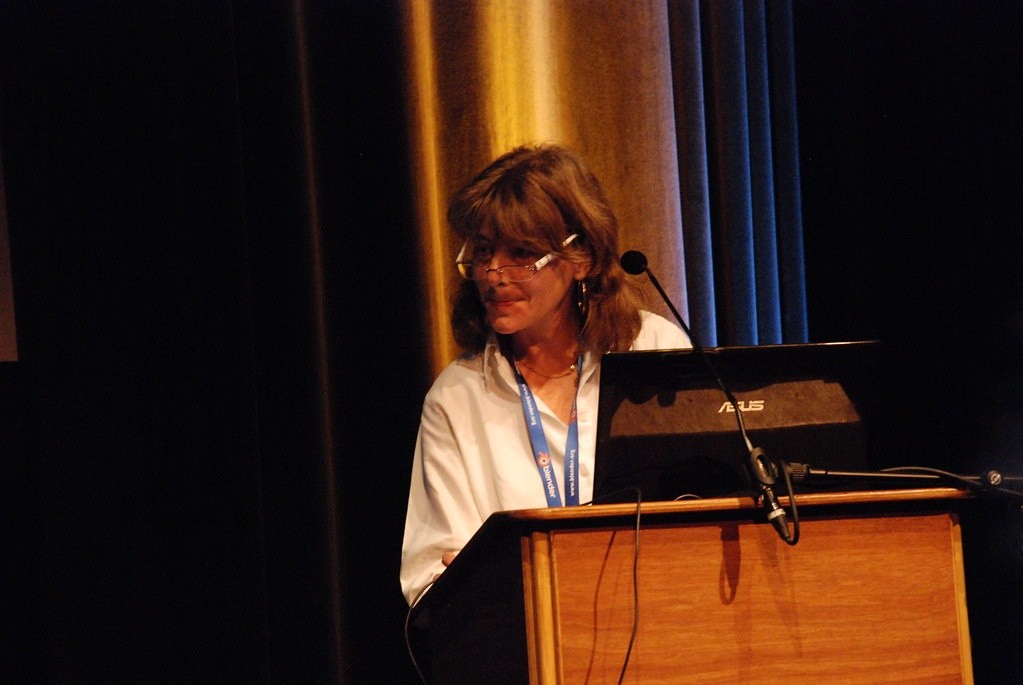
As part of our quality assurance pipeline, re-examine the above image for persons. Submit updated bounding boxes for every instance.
[399,147,693,608]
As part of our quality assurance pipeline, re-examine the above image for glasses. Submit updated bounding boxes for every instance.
[455,233,582,282]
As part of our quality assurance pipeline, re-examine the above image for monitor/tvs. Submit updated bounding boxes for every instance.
[589,339,884,507]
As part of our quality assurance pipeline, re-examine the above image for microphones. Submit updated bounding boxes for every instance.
[618,250,793,544]
